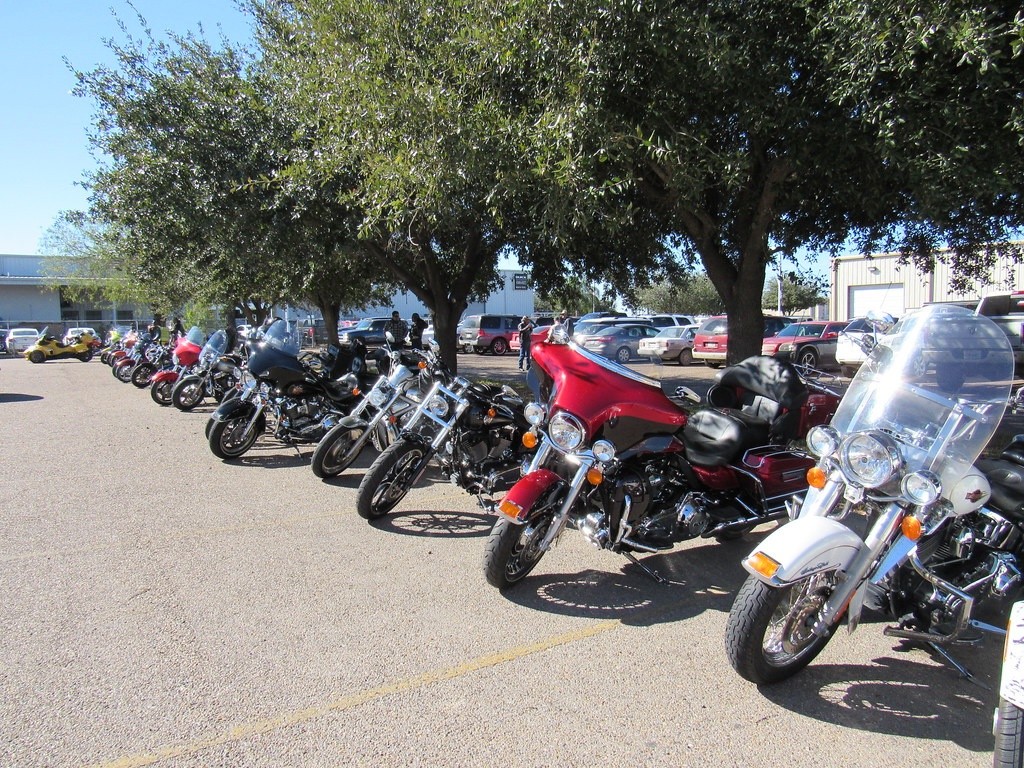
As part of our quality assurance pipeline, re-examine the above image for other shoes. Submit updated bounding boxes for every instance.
[519,368,524,372]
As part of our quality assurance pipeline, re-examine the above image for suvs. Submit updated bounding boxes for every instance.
[338,317,411,344]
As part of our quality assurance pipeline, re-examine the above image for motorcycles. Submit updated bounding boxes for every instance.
[22,326,102,363]
[95,304,1024,767]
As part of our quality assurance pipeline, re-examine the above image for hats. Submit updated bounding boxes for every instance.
[521,316,531,321]
[560,310,568,315]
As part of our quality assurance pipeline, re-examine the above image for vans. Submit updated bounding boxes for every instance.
[690,313,796,370]
[458,313,538,357]
[293,318,328,343]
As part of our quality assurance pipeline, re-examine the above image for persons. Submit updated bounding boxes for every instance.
[409,313,428,350]
[544,309,574,344]
[383,311,409,351]
[518,315,533,371]
[147,316,186,346]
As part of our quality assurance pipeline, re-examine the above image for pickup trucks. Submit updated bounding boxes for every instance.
[920,290,1024,391]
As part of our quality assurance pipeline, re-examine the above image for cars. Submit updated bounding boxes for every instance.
[834,314,929,382]
[636,324,705,366]
[1,328,41,355]
[762,321,849,376]
[583,323,673,364]
[509,325,552,350]
[60,327,103,350]
[237,323,253,339]
